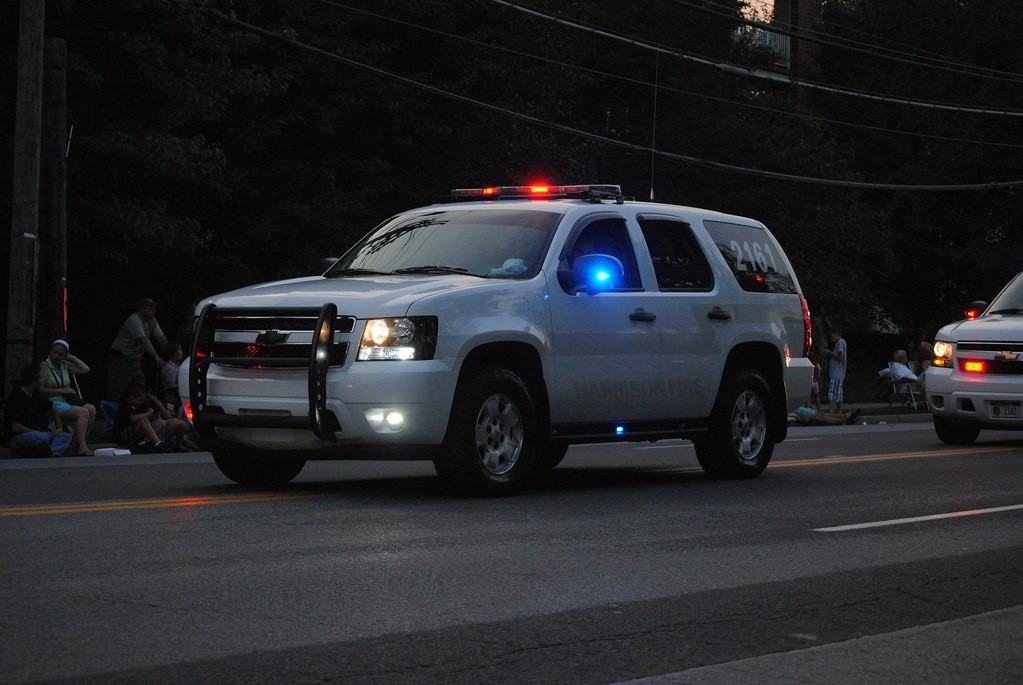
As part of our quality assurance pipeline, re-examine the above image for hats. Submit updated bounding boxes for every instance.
[139,298,159,308]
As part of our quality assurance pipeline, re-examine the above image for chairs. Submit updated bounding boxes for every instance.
[100,399,122,432]
[888,361,930,413]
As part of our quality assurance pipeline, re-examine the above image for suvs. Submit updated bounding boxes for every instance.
[177,185,816,501]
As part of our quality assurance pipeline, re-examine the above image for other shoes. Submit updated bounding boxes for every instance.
[174,446,193,453]
[846,408,861,424]
[154,439,172,453]
[136,443,154,454]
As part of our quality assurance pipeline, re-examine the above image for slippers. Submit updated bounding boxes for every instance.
[77,450,94,456]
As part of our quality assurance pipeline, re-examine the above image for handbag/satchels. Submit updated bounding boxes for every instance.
[64,393,85,406]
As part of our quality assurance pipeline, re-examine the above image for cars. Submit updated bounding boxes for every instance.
[925,271,1023,446]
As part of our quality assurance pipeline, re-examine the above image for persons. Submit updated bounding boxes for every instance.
[3,298,209,458]
[784,304,933,423]
[492,237,609,294]
